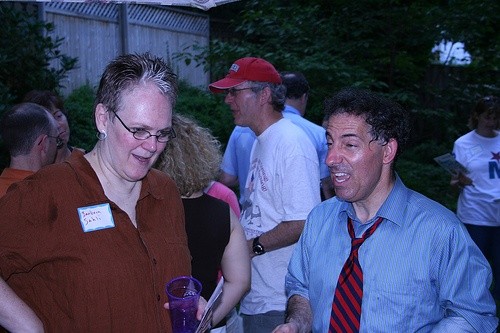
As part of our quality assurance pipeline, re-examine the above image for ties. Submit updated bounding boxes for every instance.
[328,216,383,333]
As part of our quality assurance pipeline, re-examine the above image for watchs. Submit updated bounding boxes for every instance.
[252,237,265,255]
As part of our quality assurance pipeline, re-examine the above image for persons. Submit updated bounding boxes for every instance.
[1,54,335,333]
[272,88,500,333]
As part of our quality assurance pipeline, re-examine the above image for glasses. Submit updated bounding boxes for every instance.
[37,135,63,146]
[109,106,177,143]
[478,96,500,110]
[226,86,253,95]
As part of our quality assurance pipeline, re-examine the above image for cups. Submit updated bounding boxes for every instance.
[166,276,201,333]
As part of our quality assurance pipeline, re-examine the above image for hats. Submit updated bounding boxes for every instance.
[209,57,283,94]
